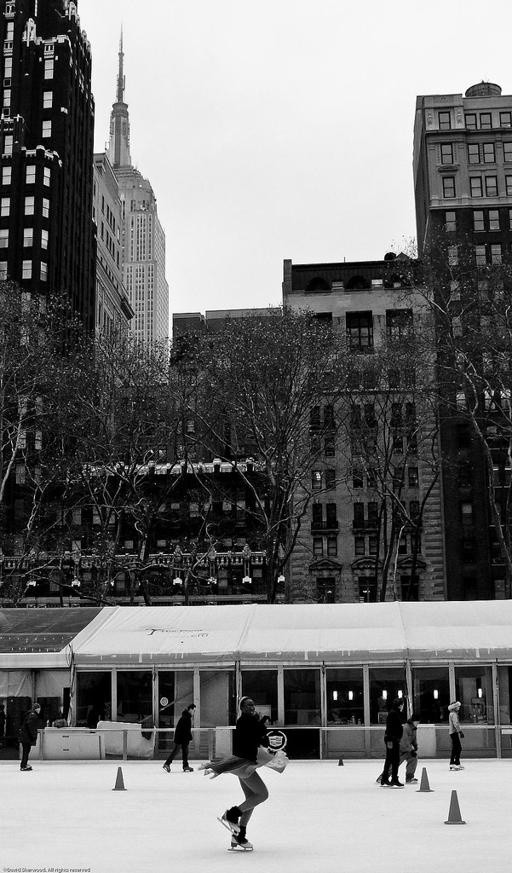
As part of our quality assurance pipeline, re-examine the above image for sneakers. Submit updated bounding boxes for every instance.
[163,763,171,771]
[449,763,455,768]
[183,765,193,770]
[20,763,32,770]
[406,777,418,783]
[454,763,464,769]
[376,776,383,783]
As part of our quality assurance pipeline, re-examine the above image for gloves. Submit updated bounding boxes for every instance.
[459,731,464,739]
[412,746,417,752]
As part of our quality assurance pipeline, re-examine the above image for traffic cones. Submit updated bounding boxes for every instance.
[443,788,466,824]
[109,764,128,790]
[414,766,436,792]
[336,753,347,766]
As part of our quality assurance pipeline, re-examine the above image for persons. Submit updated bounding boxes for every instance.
[378,697,405,787]
[369,695,379,723]
[164,702,197,771]
[310,707,335,725]
[84,695,109,729]
[376,710,420,783]
[16,702,43,769]
[327,707,343,721]
[222,695,270,849]
[446,700,465,769]
[0,703,8,739]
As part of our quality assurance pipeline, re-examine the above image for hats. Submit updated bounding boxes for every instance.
[32,703,40,710]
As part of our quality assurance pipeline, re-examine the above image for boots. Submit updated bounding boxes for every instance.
[223,806,243,833]
[390,775,405,786]
[380,773,392,785]
[230,826,254,848]
